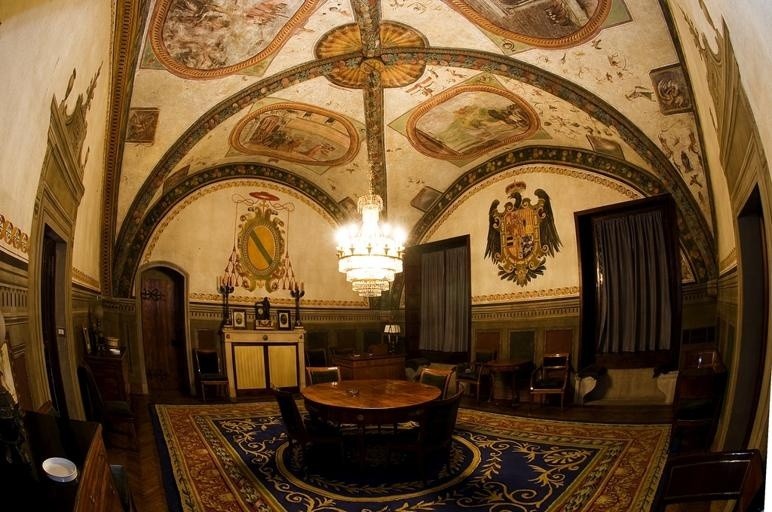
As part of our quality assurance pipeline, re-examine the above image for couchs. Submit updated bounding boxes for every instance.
[572,351,680,407]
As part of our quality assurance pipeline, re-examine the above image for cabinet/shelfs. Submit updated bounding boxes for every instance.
[660,450,766,511]
[83,346,131,414]
[0,410,127,512]
[672,351,727,453]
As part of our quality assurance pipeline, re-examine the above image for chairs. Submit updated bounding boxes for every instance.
[305,366,341,390]
[394,367,459,439]
[458,350,497,401]
[384,386,465,480]
[192,350,229,401]
[77,367,138,452]
[529,353,570,409]
[276,391,341,479]
[305,348,341,387]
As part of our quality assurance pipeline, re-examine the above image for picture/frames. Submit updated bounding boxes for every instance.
[82,328,91,353]
[233,309,246,329]
[277,310,291,330]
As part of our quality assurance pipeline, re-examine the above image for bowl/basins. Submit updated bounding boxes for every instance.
[41,455,79,482]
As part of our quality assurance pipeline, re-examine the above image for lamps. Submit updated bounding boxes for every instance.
[384,324,400,353]
[95,305,104,351]
[334,70,407,298]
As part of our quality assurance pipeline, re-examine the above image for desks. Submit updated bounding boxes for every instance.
[300,379,442,448]
[327,353,400,380]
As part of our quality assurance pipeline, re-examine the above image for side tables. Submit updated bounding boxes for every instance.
[489,357,531,407]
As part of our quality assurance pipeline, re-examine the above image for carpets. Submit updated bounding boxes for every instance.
[147,398,672,511]
